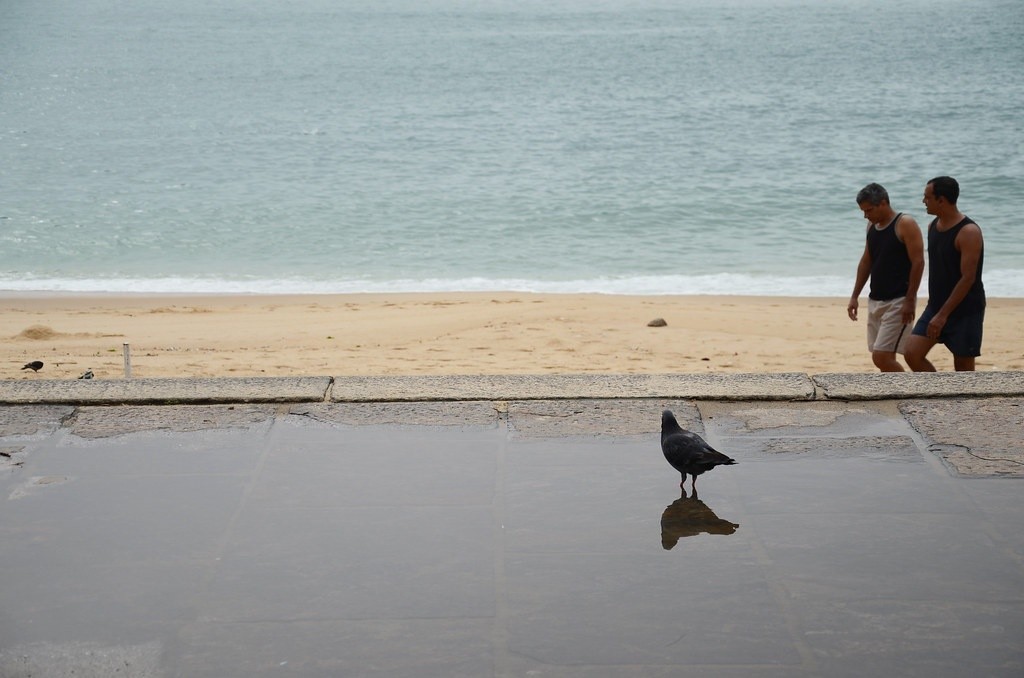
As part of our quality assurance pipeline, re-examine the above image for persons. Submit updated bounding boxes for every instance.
[846,182,925,373]
[908,175,988,371]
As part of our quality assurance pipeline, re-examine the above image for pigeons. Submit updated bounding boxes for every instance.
[21,361,44,374]
[660,409,742,492]
[77,367,95,380]
